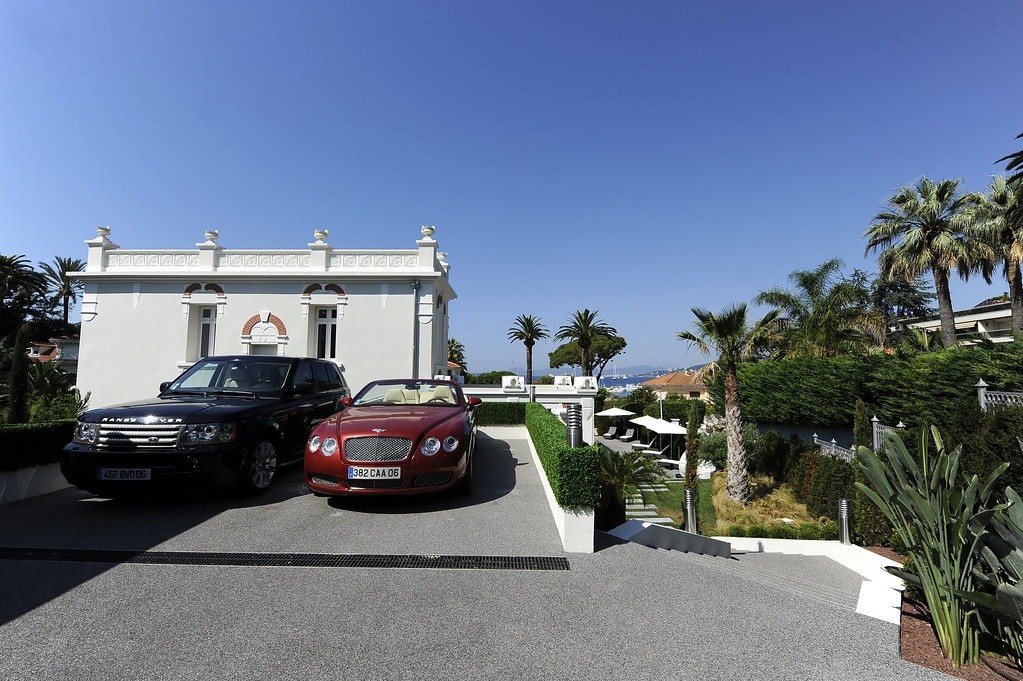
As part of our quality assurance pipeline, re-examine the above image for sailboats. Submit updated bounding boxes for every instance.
[598,365,643,392]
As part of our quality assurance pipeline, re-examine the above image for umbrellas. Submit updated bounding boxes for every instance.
[629,414,687,459]
[595,406,637,426]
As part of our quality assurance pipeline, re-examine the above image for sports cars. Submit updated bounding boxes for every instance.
[302,378,485,498]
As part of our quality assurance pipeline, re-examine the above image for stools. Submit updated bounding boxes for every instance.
[657,458,680,470]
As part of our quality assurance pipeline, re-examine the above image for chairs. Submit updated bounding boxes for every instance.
[632,437,657,450]
[602,426,617,439]
[382,388,407,405]
[619,428,635,442]
[642,445,671,459]
[430,385,457,405]
[222,369,250,389]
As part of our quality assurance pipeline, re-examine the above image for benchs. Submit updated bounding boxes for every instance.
[402,390,435,404]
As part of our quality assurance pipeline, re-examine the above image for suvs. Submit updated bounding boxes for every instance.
[59,354,354,506]
